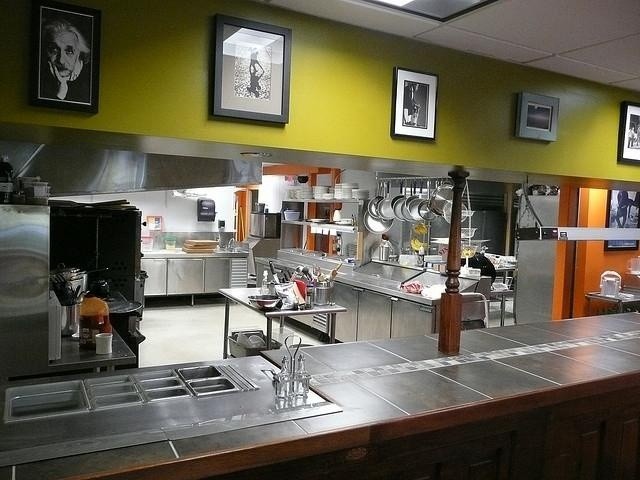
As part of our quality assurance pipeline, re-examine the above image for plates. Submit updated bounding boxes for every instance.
[346,243,357,258]
[307,218,330,223]
[286,181,369,200]
[430,238,449,245]
[423,256,446,263]
[335,217,353,226]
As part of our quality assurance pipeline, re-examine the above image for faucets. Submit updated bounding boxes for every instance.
[228,237,236,248]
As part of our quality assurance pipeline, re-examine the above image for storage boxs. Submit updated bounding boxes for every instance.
[228,333,281,358]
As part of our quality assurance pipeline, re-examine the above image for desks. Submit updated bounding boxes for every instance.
[0,319,137,383]
[494,266,516,309]
[489,289,514,327]
[0,312,640,480]
[218,286,347,359]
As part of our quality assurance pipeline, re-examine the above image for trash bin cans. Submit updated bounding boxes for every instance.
[140,271,149,309]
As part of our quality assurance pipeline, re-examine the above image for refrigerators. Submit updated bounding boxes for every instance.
[512,193,561,325]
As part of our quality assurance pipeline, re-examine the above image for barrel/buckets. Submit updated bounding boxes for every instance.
[599,269,622,296]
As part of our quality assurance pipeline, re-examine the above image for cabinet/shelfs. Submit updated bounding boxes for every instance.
[584,289,640,317]
[142,257,229,295]
[280,198,370,233]
[257,263,436,344]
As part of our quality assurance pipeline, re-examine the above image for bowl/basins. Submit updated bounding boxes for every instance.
[460,227,478,239]
[460,246,479,258]
[248,335,265,348]
[461,210,476,216]
[284,211,301,221]
[236,332,253,348]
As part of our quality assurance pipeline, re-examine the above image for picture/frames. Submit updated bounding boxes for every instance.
[604,190,639,251]
[211,13,292,124]
[515,90,560,142]
[617,100,640,166]
[390,66,439,141]
[28,0,102,113]
[146,216,162,231]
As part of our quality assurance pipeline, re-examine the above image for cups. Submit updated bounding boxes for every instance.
[626,257,640,274]
[59,301,80,335]
[314,277,335,306]
[95,333,114,356]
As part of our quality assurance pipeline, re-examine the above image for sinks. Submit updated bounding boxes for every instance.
[228,249,240,253]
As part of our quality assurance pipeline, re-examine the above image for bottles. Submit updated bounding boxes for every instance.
[304,284,316,310]
[295,354,313,396]
[260,270,271,293]
[1,155,15,206]
[273,356,293,402]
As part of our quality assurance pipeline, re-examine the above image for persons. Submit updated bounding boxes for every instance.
[628,126,640,148]
[250,51,266,72]
[403,82,422,126]
[40,18,92,103]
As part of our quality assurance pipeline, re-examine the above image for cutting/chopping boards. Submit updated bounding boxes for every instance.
[182,239,219,254]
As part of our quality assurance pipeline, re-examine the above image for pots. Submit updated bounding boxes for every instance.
[360,173,469,235]
[246,293,288,311]
[49,260,110,295]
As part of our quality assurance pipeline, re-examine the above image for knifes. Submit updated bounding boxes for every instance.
[280,269,295,285]
[268,260,281,285]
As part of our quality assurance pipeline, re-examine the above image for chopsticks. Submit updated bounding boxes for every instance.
[48,273,83,305]
